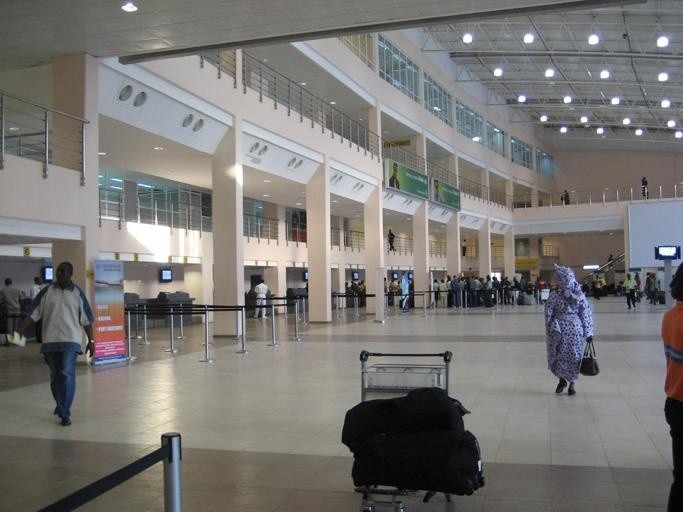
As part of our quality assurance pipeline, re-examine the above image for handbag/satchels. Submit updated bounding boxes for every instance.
[341,388,484,495]
[580,357,599,375]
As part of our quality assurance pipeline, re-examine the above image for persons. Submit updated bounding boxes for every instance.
[384,276,414,308]
[433,179,443,203]
[387,228,396,252]
[640,176,649,199]
[388,162,399,189]
[346,281,366,306]
[29,276,49,343]
[582,255,613,300]
[433,274,553,307]
[542,264,599,397]
[462,238,466,256]
[254,279,268,319]
[14,262,95,427]
[0,278,25,347]
[560,189,570,205]
[660,260,683,512]
[623,272,657,309]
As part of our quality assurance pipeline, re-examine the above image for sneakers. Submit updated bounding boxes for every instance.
[556,378,567,392]
[569,383,576,394]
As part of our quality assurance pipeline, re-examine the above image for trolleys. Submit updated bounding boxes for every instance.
[352,350,454,511]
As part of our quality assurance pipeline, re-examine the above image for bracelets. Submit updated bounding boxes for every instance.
[89,339,94,343]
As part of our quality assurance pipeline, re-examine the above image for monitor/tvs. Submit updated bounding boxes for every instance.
[392,272,398,280]
[41,265,53,284]
[159,268,172,282]
[303,272,308,281]
[353,272,359,280]
[408,272,412,280]
[655,245,681,260]
